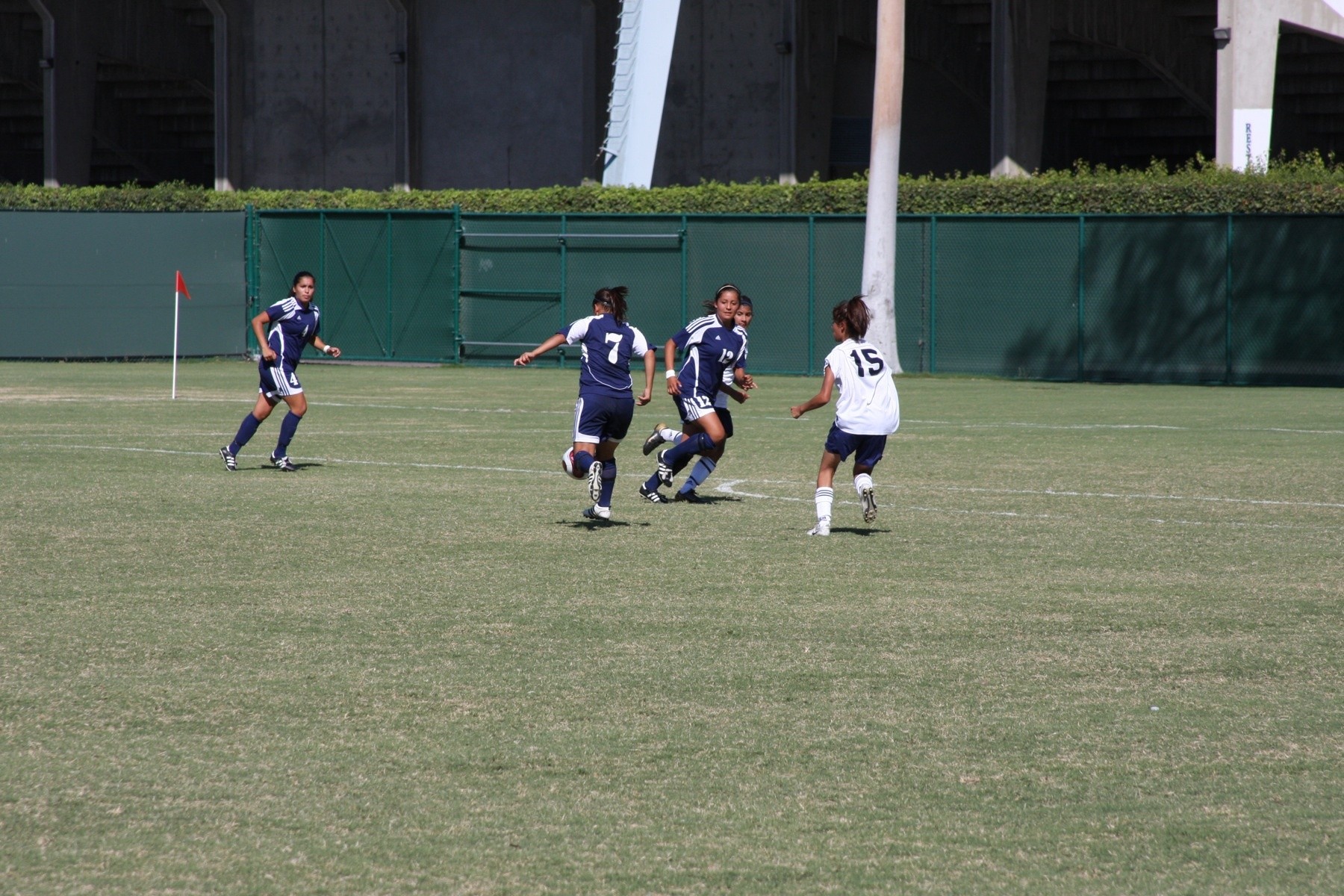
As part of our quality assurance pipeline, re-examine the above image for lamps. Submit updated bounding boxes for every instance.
[1213,27,1231,39]
[389,51,405,63]
[775,40,793,55]
[39,56,54,68]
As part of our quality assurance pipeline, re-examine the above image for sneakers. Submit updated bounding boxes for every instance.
[582,502,611,521]
[655,448,674,488]
[221,445,238,473]
[675,488,712,504]
[644,423,669,456]
[808,522,831,537]
[588,461,603,501]
[639,481,668,505]
[271,450,296,471]
[860,484,878,523]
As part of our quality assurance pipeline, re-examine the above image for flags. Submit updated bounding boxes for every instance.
[175,270,191,299]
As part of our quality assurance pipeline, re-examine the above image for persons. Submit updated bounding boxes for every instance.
[219,271,341,473]
[514,286,657,520]
[790,295,900,537]
[514,283,758,522]
